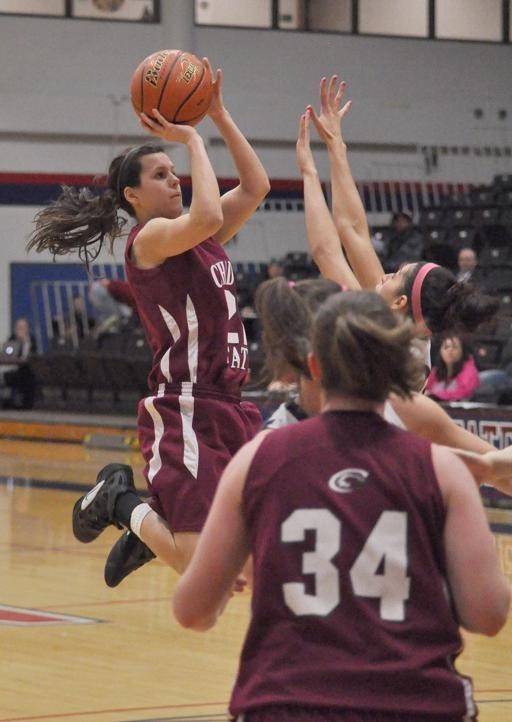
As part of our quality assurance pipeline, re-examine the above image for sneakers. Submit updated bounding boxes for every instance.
[72,463,137,543]
[104,529,158,588]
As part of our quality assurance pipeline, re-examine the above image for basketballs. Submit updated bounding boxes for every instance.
[130,48,212,125]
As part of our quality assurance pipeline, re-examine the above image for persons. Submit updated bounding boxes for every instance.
[425,329,472,397]
[254,269,510,488]
[22,54,269,586]
[168,299,509,721]
[383,205,426,272]
[52,293,93,337]
[446,238,487,286]
[234,286,259,346]
[5,318,36,362]
[294,58,493,382]
